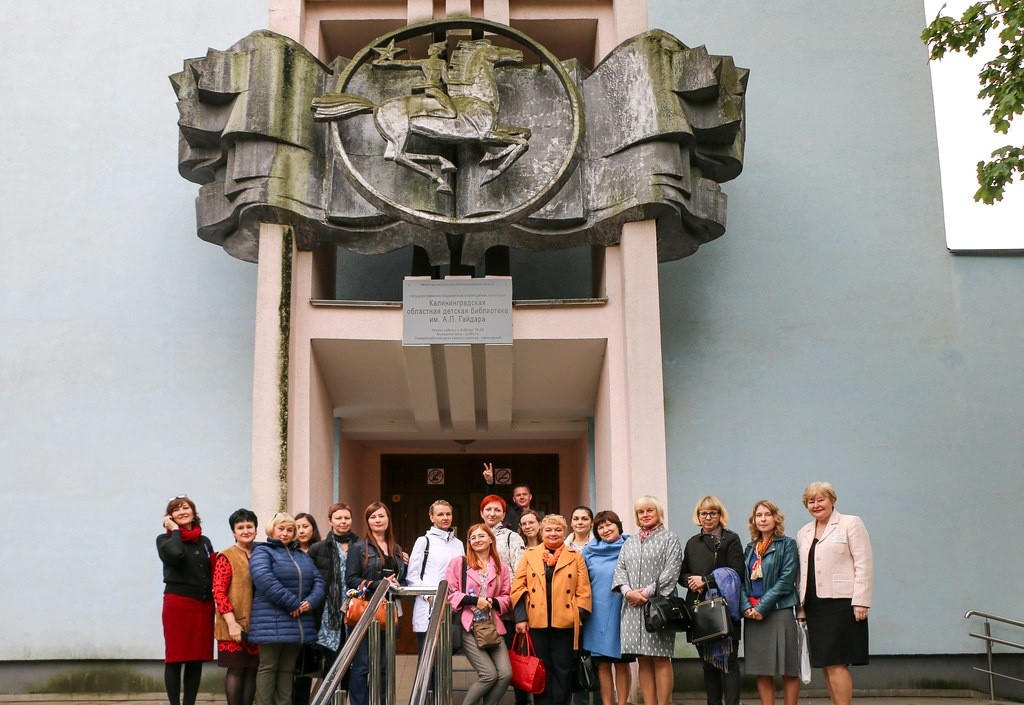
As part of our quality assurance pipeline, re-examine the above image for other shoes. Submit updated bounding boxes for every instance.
[451,611,463,656]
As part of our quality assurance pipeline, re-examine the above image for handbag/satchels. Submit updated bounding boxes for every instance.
[644,579,694,633]
[399,536,430,608]
[507,630,547,695]
[472,619,501,650]
[692,576,734,645]
[571,655,598,693]
[345,581,399,630]
[795,620,812,686]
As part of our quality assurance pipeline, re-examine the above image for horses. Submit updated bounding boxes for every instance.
[310,39,531,193]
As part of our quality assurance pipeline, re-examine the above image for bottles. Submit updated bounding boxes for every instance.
[468,589,487,619]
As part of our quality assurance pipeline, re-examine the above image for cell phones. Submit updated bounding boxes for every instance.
[381,569,393,578]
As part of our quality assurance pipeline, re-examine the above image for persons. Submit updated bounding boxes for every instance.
[309,503,409,699]
[345,502,405,705]
[741,499,800,705]
[445,524,512,705]
[518,510,543,550]
[678,495,744,705]
[249,514,324,705]
[406,501,464,705]
[611,496,682,705]
[156,497,214,705]
[582,511,630,705]
[480,495,531,705]
[292,513,321,705]
[563,506,603,705]
[372,41,475,118]
[213,509,259,705]
[511,514,592,705]
[797,482,873,705]
[483,462,545,533]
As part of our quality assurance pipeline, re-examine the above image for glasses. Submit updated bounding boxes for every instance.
[168,494,187,503]
[468,534,486,542]
[697,512,720,518]
